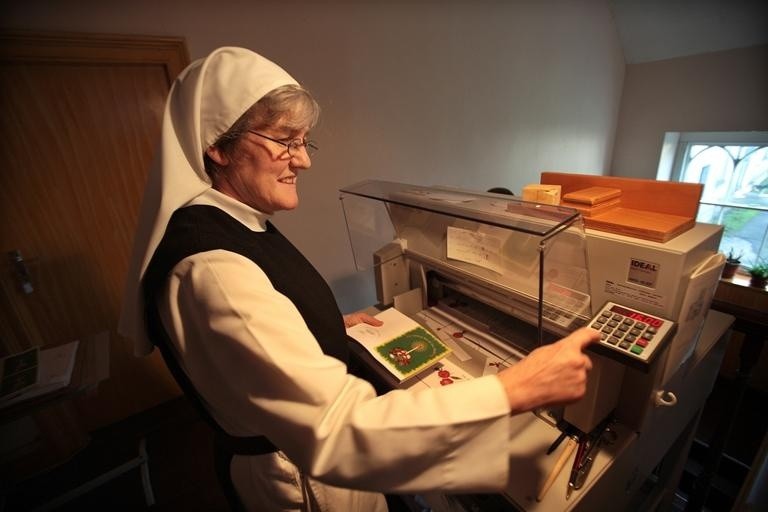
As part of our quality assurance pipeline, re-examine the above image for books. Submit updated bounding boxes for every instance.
[1,344,44,401]
[1,339,82,408]
[344,306,453,384]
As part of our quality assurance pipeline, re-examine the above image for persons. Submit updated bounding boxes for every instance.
[116,46,607,511]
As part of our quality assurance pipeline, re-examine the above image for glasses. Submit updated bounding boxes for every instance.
[248,131,320,161]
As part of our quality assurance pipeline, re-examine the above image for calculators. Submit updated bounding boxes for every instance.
[586,301,677,363]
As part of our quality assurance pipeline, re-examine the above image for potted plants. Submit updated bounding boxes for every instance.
[724,248,768,291]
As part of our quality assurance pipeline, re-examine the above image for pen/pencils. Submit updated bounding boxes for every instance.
[536,424,619,502]
